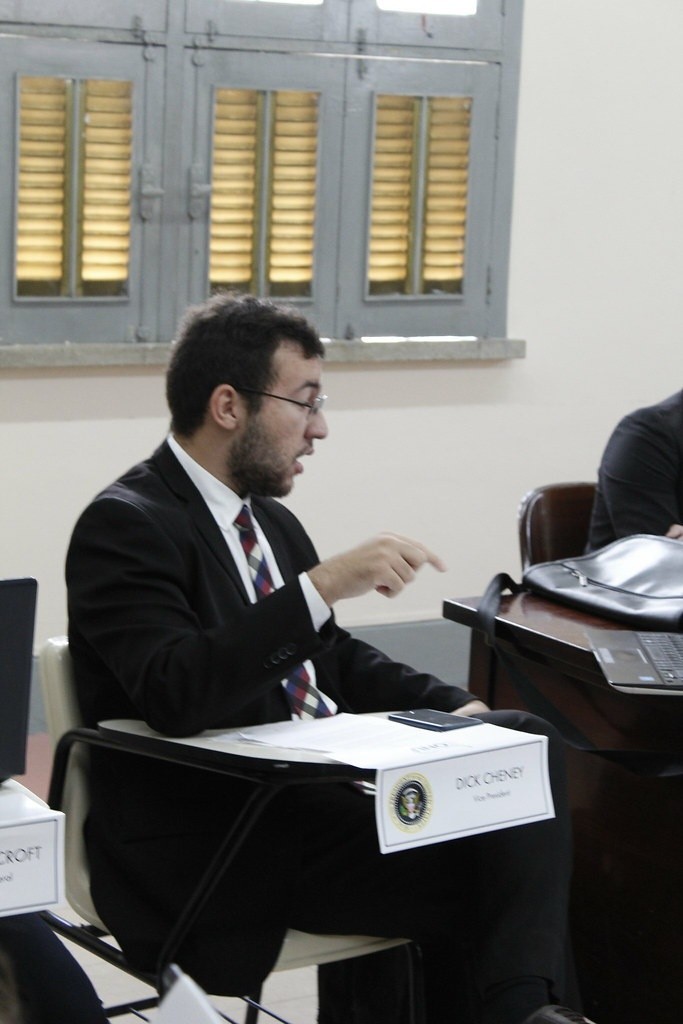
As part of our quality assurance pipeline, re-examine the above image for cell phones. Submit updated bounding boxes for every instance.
[388,707,483,733]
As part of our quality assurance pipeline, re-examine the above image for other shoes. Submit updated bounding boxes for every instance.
[522,1005,598,1024]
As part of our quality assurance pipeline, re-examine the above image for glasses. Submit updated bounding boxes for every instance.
[233,384,328,415]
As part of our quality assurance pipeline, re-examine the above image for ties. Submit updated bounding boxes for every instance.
[232,504,334,726]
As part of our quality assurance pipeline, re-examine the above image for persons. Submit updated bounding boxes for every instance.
[62,293,573,1024]
[581,388,683,554]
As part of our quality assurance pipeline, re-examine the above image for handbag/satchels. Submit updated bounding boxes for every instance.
[523,534,683,633]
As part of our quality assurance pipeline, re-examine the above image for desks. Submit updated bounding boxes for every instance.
[442,591,682,1022]
[45,704,409,972]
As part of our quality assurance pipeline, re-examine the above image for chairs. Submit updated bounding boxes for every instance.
[0,579,161,1024]
[520,483,598,576]
[37,636,436,1024]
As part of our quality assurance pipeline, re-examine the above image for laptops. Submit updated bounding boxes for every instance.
[583,625,683,696]
